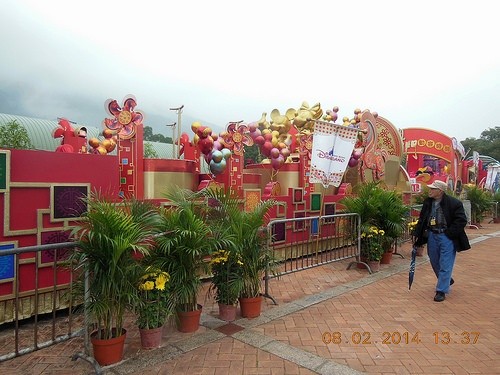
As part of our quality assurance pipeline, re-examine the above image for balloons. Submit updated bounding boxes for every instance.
[363,109,378,118]
[260,141,272,157]
[192,122,212,138]
[342,118,356,129]
[55,145,73,153]
[353,150,362,159]
[353,109,362,123]
[204,148,232,174]
[323,106,339,122]
[269,148,290,169]
[190,134,199,146]
[348,157,358,167]
[89,138,99,147]
[249,123,271,143]
[96,128,117,154]
[200,134,225,153]
[219,131,228,137]
[271,132,287,150]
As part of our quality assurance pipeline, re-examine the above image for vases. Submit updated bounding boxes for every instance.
[416,245,424,257]
[137,323,163,350]
[368,262,382,272]
[217,301,237,323]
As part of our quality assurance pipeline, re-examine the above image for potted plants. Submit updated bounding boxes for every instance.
[327,177,412,269]
[463,182,500,229]
[56,184,287,367]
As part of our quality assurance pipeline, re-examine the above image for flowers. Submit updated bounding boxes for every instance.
[135,265,174,330]
[359,224,386,262]
[407,218,427,248]
[209,248,243,304]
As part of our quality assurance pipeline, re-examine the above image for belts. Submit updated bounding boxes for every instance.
[427,226,447,234]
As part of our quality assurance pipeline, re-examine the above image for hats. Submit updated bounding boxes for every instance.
[427,179,447,191]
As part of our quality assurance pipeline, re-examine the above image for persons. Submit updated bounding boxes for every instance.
[414,180,471,301]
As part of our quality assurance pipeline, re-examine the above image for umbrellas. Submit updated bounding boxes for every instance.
[409,245,416,291]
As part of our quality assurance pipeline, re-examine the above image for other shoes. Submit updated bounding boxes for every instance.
[450,277,454,285]
[434,291,445,302]
